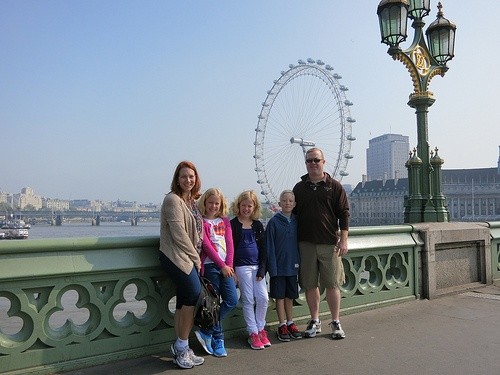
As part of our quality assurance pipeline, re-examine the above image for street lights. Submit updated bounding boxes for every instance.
[376,1,456,224]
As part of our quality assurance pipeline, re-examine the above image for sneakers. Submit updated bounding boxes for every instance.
[194,328,214,355]
[248,330,271,349]
[277,323,302,342]
[212,338,228,357]
[169,343,204,368]
[330,321,345,340]
[304,319,321,337]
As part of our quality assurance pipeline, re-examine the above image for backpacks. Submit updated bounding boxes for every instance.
[193,274,221,330]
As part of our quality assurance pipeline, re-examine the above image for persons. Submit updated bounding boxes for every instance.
[292,147,351,339]
[194,189,239,356]
[230,190,272,349]
[266,189,303,342]
[160,161,205,368]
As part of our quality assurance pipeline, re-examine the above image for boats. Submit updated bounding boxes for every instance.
[0,214,32,239]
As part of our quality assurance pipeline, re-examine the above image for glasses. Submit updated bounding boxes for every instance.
[306,158,323,163]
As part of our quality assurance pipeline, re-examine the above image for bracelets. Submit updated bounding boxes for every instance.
[195,261,201,267]
